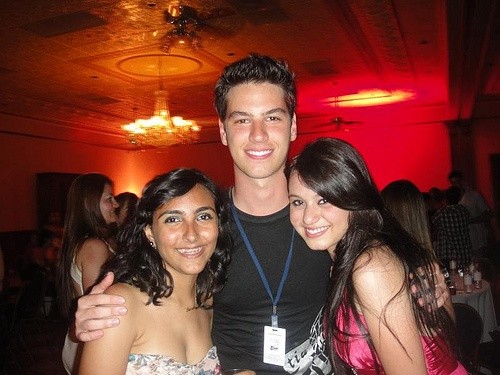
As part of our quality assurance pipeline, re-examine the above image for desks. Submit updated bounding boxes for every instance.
[451,279,497,343]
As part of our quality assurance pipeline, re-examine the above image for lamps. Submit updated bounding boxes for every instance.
[119,57,201,149]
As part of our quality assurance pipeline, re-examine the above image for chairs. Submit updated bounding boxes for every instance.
[452,303,483,356]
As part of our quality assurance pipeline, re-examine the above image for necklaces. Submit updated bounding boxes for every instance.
[172,281,196,312]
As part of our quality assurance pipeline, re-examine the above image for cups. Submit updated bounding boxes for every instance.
[444,258,483,295]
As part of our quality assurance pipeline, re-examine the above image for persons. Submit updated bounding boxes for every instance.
[61,173,140,375]
[378,170,500,260]
[284,136,470,375]
[77,168,256,375]
[63,52,455,375]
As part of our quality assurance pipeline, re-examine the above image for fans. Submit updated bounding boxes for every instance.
[312,96,364,127]
